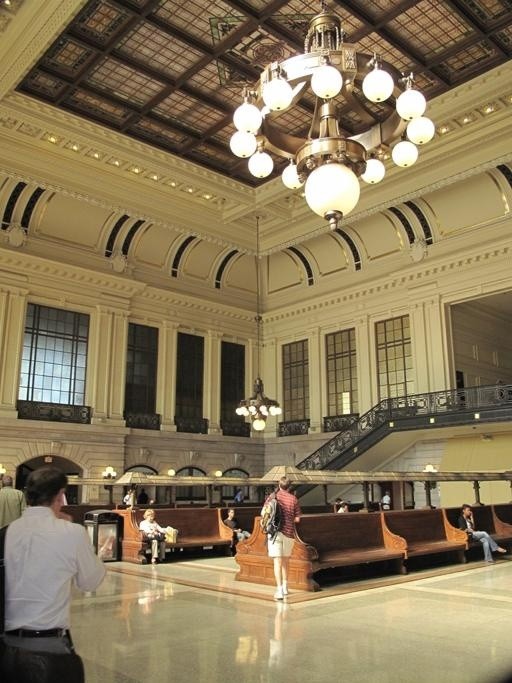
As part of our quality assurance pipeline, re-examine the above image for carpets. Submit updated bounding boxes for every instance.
[103,553,511,604]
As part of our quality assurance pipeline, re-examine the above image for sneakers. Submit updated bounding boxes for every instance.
[152,558,166,564]
[276,585,287,600]
[497,548,506,553]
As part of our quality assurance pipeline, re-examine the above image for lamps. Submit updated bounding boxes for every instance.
[103,465,116,503]
[235,213,283,431]
[423,464,436,504]
[215,470,223,477]
[168,469,175,477]
[228,0,436,231]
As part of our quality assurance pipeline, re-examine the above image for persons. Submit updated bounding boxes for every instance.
[139,507,168,564]
[123,488,132,505]
[334,497,342,512]
[458,503,508,562]
[259,474,302,600]
[1,473,29,529]
[380,490,391,510]
[138,487,149,503]
[234,486,246,502]
[225,507,252,542]
[1,465,108,681]
[129,489,137,508]
[337,501,349,513]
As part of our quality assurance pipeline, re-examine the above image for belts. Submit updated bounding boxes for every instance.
[7,629,59,638]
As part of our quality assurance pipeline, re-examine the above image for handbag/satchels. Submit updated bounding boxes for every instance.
[166,527,177,543]
[467,532,473,542]
[0,639,84,683]
[146,531,165,541]
[261,493,283,537]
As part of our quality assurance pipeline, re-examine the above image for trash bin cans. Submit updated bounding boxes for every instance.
[84,509,122,561]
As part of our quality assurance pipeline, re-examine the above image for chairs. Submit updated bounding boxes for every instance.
[230,504,510,590]
[116,504,407,565]
